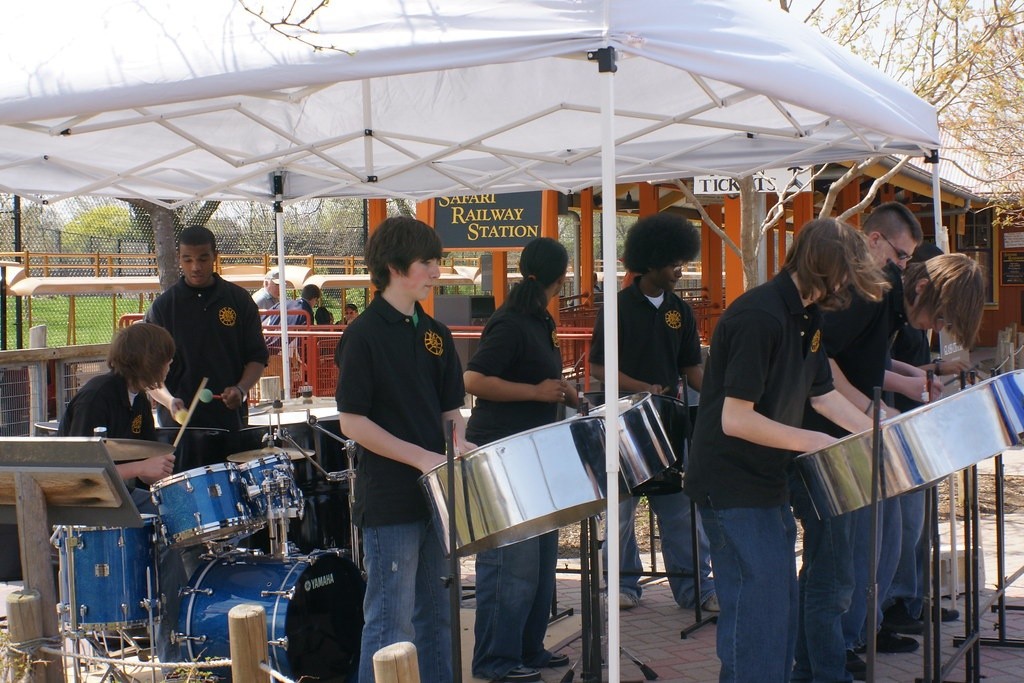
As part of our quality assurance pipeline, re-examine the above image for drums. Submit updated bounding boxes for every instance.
[791,367,1024,522]
[416,391,705,561]
[236,451,305,521]
[51,522,164,638]
[175,544,367,683]
[148,461,268,549]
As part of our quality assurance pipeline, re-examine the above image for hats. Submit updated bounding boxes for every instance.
[265,268,285,284]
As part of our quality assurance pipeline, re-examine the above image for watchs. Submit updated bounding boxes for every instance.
[234,385,248,404]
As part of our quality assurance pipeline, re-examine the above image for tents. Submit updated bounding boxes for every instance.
[0,0,940,683]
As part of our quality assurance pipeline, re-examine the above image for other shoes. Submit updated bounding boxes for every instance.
[704,595,719,610]
[884,605,923,634]
[844,649,867,679]
[604,594,637,607]
[919,601,958,620]
[875,632,918,653]
[506,666,540,681]
[546,653,569,666]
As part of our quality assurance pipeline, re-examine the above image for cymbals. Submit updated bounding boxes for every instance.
[93,436,177,461]
[244,395,340,427]
[226,446,316,463]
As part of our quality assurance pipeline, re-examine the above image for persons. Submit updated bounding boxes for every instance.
[251,266,358,362]
[591,214,721,615]
[684,206,987,683]
[335,217,480,683]
[144,225,270,474]
[56,323,175,486]
[463,242,579,683]
[592,272,602,292]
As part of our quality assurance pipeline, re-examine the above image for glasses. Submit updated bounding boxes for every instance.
[879,233,913,261]
[669,263,683,272]
[932,312,954,327]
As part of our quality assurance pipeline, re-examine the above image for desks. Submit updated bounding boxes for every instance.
[33,396,340,548]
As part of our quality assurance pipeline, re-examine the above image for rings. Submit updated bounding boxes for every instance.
[563,392,565,397]
